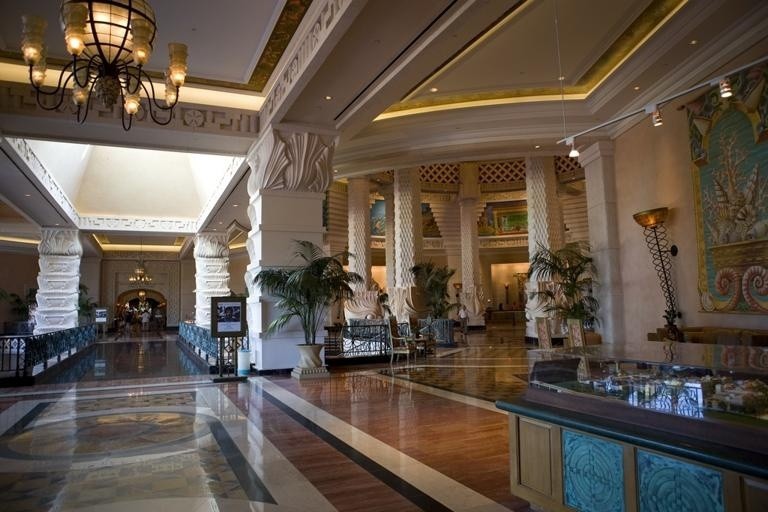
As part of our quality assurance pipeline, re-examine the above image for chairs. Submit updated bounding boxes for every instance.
[385,314,437,366]
[417,316,455,349]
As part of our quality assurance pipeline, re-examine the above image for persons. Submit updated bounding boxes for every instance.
[139,310,151,331]
[458,304,470,335]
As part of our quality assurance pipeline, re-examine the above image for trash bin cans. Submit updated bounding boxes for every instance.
[237,350,250,374]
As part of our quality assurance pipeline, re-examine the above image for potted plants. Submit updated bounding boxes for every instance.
[251,236,366,368]
[1,287,39,335]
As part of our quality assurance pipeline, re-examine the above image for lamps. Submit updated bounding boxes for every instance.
[18,0,191,131]
[718,78,732,98]
[651,108,663,126]
[128,238,154,286]
[632,205,681,338]
[568,142,579,158]
